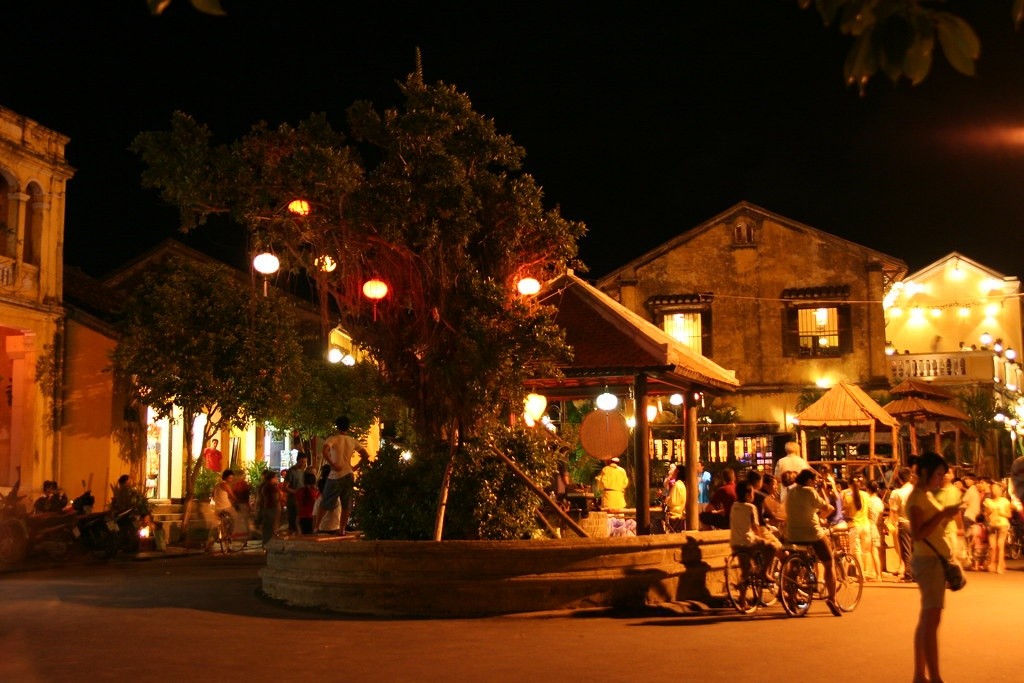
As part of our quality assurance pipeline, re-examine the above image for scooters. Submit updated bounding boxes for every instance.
[1,464,150,567]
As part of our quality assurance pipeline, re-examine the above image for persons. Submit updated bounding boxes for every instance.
[1012,435,1024,506]
[313,416,370,535]
[705,468,736,518]
[933,335,943,352]
[551,441,570,495]
[730,469,783,611]
[30,480,58,515]
[232,469,252,548]
[203,438,222,472]
[596,457,629,522]
[893,350,909,354]
[958,341,977,352]
[283,452,331,534]
[255,469,282,548]
[774,442,823,481]
[904,451,960,683]
[781,469,842,616]
[280,469,288,478]
[660,458,711,533]
[818,454,917,583]
[941,469,1011,575]
[205,468,239,552]
[111,474,134,530]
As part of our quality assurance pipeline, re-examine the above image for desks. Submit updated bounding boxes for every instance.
[601,506,663,519]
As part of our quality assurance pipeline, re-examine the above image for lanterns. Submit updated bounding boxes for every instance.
[328,345,355,366]
[597,382,684,427]
[518,271,541,306]
[253,247,279,298]
[363,277,388,322]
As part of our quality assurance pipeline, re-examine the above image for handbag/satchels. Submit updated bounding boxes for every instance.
[946,561,966,592]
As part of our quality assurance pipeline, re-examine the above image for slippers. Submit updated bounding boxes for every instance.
[825,599,841,616]
[788,599,801,614]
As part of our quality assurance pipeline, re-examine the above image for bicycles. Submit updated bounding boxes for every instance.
[217,500,249,555]
[778,517,865,618]
[724,532,792,615]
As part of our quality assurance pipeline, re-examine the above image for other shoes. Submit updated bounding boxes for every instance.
[737,600,752,609]
[756,573,776,583]
